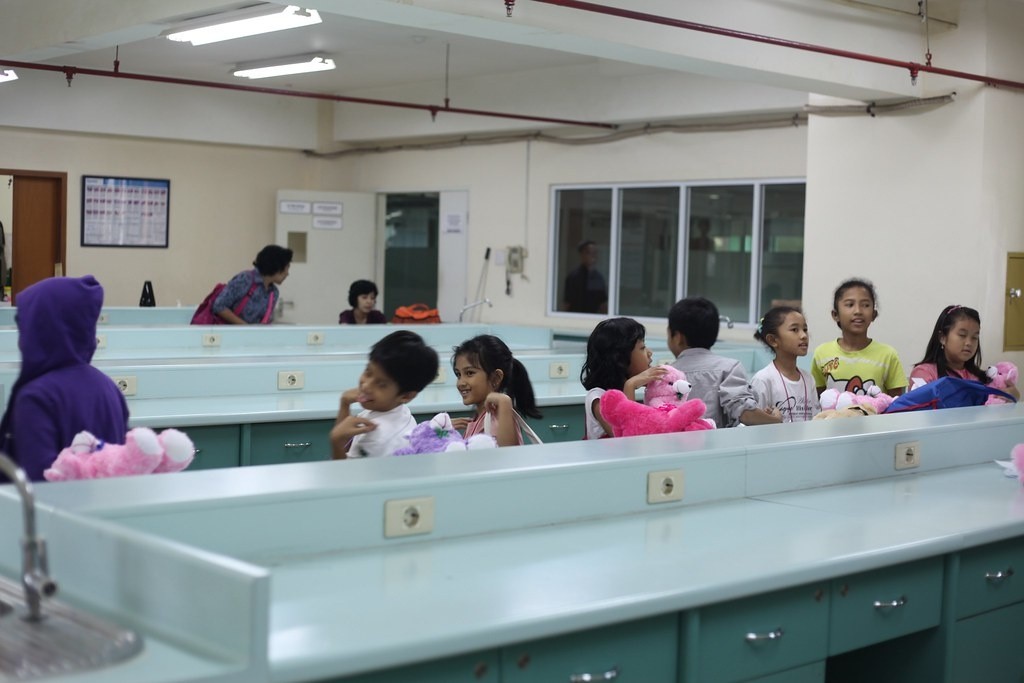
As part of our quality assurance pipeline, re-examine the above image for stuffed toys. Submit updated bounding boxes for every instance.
[1014,443,1024,487]
[600,389,716,437]
[812,403,878,419]
[819,385,898,414]
[393,412,497,457]
[984,362,1017,406]
[644,364,692,410]
[42,426,194,482]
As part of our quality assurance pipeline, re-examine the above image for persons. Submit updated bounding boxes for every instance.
[213,244,293,326]
[448,334,543,447]
[907,305,1020,404]
[580,317,669,438]
[329,329,440,459]
[667,297,782,428]
[339,280,387,324]
[749,305,822,422]
[0,274,129,485]
[811,280,910,398]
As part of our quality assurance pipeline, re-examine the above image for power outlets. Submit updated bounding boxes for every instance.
[382,498,434,537]
[96,334,107,348]
[894,441,921,469]
[201,334,221,347]
[549,363,570,379]
[647,469,683,504]
[307,332,325,345]
[658,358,673,368]
[108,375,137,396]
[432,365,448,383]
[97,315,107,324]
[278,371,303,389]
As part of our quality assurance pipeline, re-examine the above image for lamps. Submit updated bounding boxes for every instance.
[0,66,18,83]
[158,5,323,46]
[231,52,336,80]
[460,299,492,323]
[718,314,733,329]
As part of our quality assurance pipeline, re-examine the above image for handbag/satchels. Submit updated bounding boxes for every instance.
[190,270,255,325]
[880,376,1017,414]
[392,303,441,323]
[139,281,155,307]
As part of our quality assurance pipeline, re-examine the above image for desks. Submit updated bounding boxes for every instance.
[0,302,1024,683]
